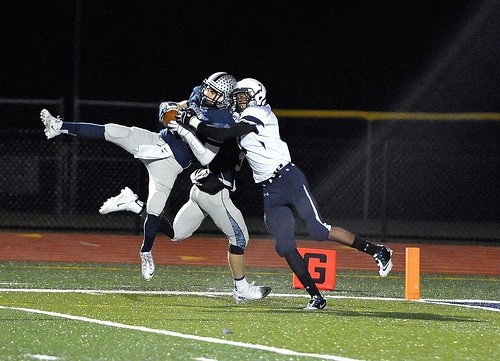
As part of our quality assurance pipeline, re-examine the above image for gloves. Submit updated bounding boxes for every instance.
[175,107,199,127]
[159,101,177,121]
[167,120,183,136]
[190,164,212,186]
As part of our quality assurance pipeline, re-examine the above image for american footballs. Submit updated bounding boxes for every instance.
[160,108,179,128]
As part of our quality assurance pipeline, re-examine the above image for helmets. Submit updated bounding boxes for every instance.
[203,72,237,101]
[231,78,266,106]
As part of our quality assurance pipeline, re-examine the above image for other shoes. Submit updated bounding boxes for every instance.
[99,187,138,214]
[233,280,272,305]
[303,294,327,310]
[373,243,394,277]
[40,109,62,139]
[139,240,155,282]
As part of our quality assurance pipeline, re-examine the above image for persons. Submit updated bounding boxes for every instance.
[39,71,393,310]
